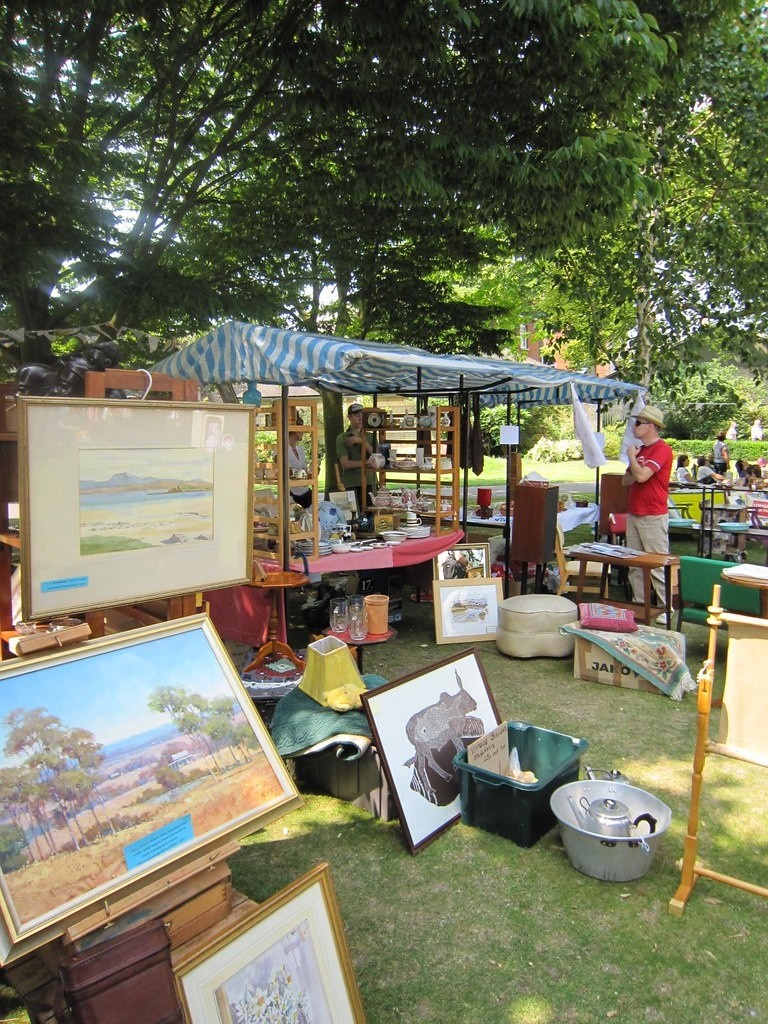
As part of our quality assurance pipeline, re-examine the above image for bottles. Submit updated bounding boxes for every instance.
[389,410,394,423]
[401,408,414,429]
[417,416,432,428]
[494,516,514,523]
[369,452,385,469]
[442,411,450,427]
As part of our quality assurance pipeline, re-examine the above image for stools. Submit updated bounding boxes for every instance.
[497,594,578,659]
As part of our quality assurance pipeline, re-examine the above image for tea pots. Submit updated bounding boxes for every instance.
[584,765,631,785]
[566,793,658,838]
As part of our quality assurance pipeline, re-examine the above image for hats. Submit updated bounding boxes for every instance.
[348,403,363,414]
[631,405,666,429]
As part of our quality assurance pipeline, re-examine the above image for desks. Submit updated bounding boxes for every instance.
[571,548,679,630]
[201,524,465,647]
[445,501,602,558]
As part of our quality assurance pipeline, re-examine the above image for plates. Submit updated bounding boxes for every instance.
[297,539,332,556]
[349,539,400,551]
[398,526,431,538]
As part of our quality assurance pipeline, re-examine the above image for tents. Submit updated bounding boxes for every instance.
[137,321,647,600]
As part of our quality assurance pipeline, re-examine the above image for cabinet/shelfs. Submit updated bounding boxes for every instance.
[252,400,319,564]
[668,482,768,566]
[360,406,460,536]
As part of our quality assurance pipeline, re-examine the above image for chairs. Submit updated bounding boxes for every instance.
[554,523,612,598]
[677,556,768,633]
[666,496,694,520]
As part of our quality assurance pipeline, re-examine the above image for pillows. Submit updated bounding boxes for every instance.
[577,601,639,632]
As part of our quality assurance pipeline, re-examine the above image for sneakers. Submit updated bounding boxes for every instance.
[655,608,674,624]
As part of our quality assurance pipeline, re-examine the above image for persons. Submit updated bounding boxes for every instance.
[622,405,675,625]
[676,419,768,484]
[336,403,377,519]
[271,414,320,510]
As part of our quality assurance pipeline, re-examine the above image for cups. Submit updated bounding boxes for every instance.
[406,512,422,527]
[364,595,390,635]
[350,604,369,640]
[388,449,397,468]
[349,595,364,606]
[381,443,391,465]
[330,597,349,633]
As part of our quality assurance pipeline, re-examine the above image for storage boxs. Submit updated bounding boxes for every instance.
[574,624,686,695]
[452,721,589,849]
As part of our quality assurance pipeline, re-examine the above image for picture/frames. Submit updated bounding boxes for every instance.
[359,644,502,858]
[172,862,367,1024]
[16,394,258,621]
[433,542,492,580]
[432,577,504,644]
[0,613,304,968]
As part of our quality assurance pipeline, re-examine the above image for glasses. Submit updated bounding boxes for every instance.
[636,420,656,427]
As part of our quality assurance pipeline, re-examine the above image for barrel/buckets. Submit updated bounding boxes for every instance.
[549,767,672,883]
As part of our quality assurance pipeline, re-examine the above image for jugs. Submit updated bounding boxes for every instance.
[368,485,423,508]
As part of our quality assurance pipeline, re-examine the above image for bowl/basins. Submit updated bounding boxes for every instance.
[331,543,351,554]
[378,530,410,542]
[717,522,751,531]
[669,519,697,527]
